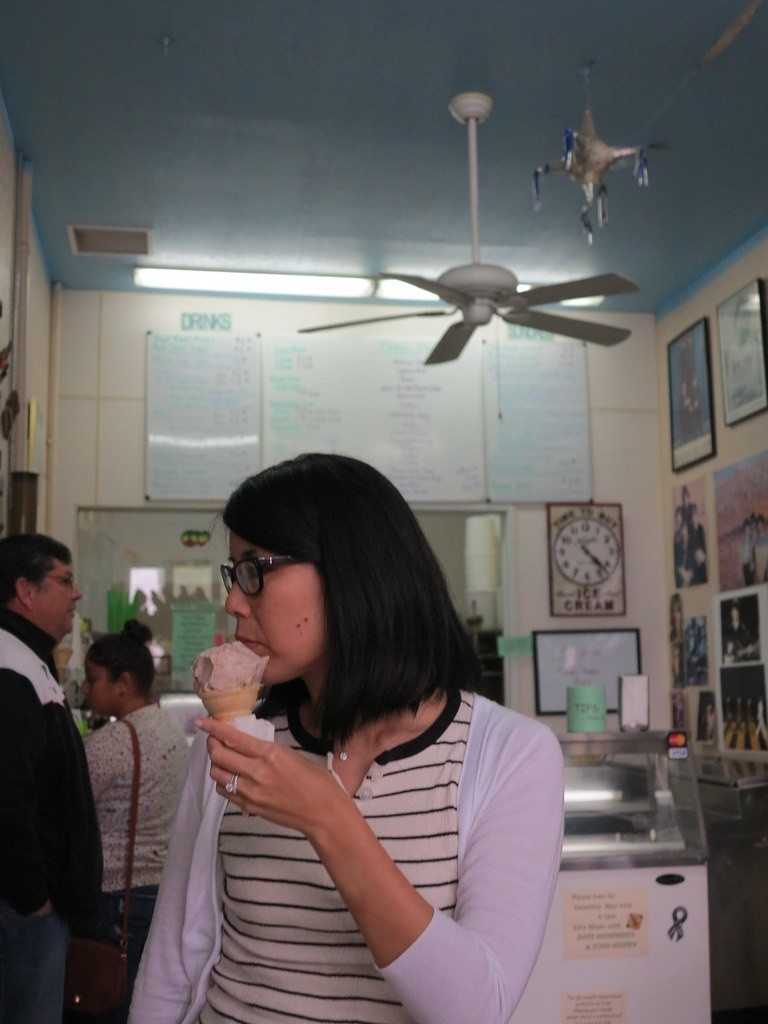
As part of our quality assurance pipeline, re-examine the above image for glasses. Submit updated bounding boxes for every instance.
[220,555,306,595]
[40,573,71,588]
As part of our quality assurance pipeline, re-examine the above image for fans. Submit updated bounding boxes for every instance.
[296,92,639,365]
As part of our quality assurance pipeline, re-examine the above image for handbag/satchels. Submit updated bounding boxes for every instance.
[63,938,128,1015]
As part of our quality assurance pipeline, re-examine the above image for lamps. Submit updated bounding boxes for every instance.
[532,65,647,245]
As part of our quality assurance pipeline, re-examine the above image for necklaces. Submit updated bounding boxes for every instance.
[339,705,430,761]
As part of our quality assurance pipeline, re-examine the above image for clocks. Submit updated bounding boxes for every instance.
[547,501,626,617]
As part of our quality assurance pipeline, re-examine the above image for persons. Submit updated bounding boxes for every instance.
[673,484,709,588]
[702,699,716,741]
[741,512,768,586]
[0,531,105,1024]
[677,340,705,435]
[724,604,760,663]
[127,452,565,1024]
[70,619,191,1024]
[726,295,763,409]
[672,693,685,729]
[723,694,768,751]
[669,593,707,690]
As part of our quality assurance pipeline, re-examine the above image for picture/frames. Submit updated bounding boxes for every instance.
[668,317,717,473]
[716,278,768,427]
[533,627,642,716]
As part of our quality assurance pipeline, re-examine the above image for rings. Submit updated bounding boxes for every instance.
[224,773,240,797]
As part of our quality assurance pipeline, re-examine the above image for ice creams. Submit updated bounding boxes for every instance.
[190,640,270,722]
